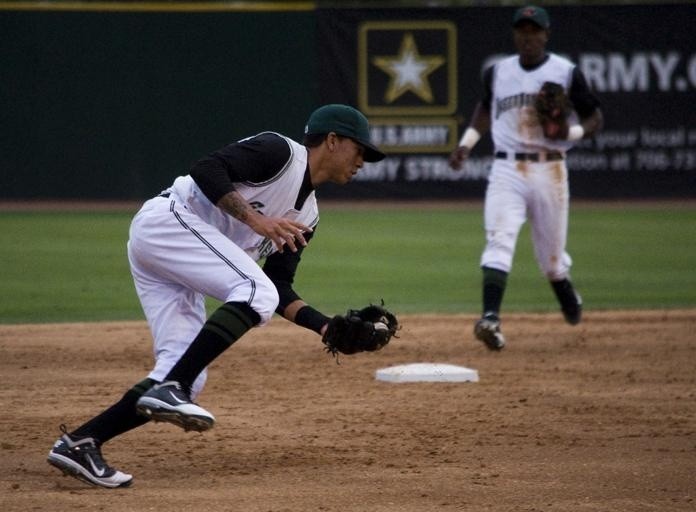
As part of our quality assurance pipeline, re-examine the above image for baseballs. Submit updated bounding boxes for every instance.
[374,322,388,332]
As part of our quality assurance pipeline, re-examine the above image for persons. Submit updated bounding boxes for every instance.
[448,6,603,350]
[45,103,398,491]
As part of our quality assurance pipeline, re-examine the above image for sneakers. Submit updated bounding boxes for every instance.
[550,277,583,326]
[136,380,216,433]
[46,424,133,489]
[473,311,506,351]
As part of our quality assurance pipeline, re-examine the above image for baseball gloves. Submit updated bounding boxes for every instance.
[324,308,398,354]
[535,81,569,142]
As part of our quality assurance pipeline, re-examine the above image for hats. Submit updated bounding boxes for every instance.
[304,104,387,163]
[513,6,550,28]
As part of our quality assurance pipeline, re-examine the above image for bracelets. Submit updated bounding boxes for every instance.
[459,125,483,151]
[566,124,585,142]
[294,303,330,334]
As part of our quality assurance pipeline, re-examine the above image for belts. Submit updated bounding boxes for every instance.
[497,151,564,162]
[160,192,187,209]
